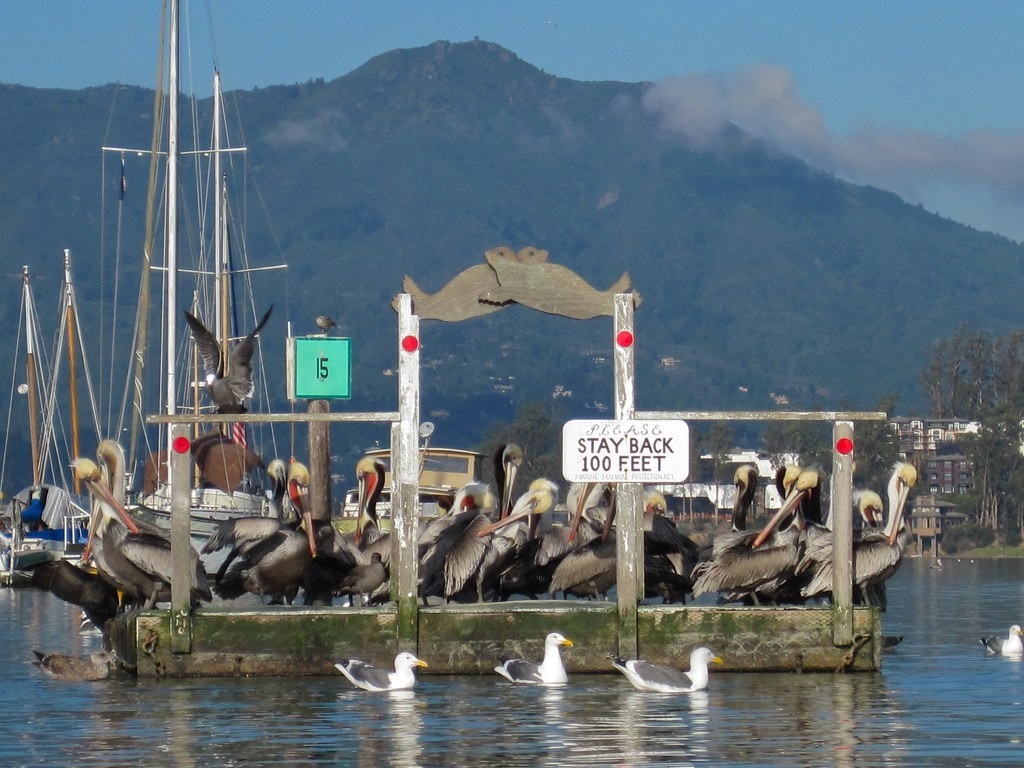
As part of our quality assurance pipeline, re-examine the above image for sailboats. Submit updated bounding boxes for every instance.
[0,1,903,683]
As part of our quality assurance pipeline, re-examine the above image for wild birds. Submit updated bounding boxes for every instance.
[67,439,920,601]
[332,652,429,692]
[881,634,905,650]
[574,660,576,662]
[606,647,723,693]
[182,303,276,413]
[978,624,1024,657]
[316,315,339,334]
[30,649,119,682]
[493,632,576,687]
